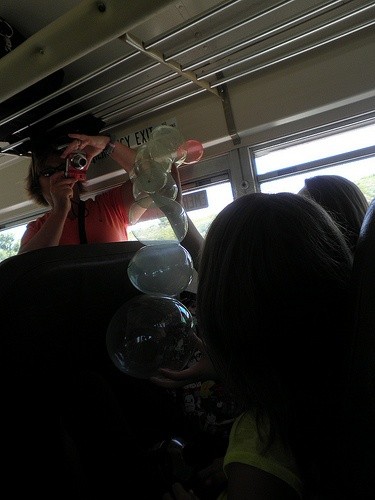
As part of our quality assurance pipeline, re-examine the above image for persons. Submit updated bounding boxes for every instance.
[152,156,370,409]
[162,190,375,500]
[14,127,166,259]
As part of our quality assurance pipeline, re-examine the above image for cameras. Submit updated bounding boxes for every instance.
[65,150,88,182]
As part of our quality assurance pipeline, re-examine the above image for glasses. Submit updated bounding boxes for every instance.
[39,165,66,176]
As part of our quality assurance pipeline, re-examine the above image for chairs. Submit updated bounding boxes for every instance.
[0,241,159,500]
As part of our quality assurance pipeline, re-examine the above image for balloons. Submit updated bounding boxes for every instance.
[99,122,210,383]
